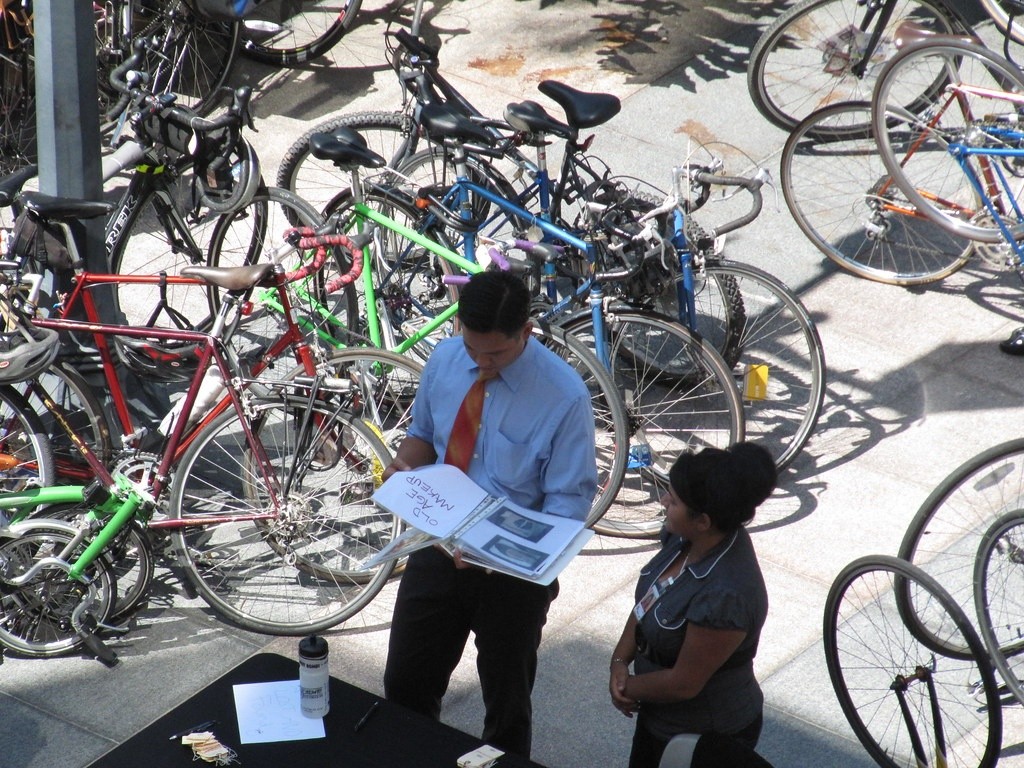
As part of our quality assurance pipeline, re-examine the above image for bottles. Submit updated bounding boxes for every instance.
[299,634,331,719]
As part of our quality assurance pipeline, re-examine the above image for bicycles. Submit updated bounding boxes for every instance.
[0,0,1023,768]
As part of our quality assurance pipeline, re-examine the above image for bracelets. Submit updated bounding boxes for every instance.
[610,659,628,673]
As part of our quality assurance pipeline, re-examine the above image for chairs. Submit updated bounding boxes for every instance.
[659,734,775,768]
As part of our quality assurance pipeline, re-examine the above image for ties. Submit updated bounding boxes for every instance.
[444,365,498,475]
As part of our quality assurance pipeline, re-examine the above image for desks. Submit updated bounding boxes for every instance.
[83,653,546,768]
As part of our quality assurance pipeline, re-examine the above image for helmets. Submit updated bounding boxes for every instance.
[0,326,60,385]
[113,335,213,383]
[193,126,261,214]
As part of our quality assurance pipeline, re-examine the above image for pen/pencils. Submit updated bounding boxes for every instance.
[353,699,380,733]
[168,719,215,743]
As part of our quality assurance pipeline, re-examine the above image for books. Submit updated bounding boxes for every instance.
[370,465,595,586]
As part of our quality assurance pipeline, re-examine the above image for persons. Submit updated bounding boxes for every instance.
[610,442,777,768]
[381,271,598,760]
